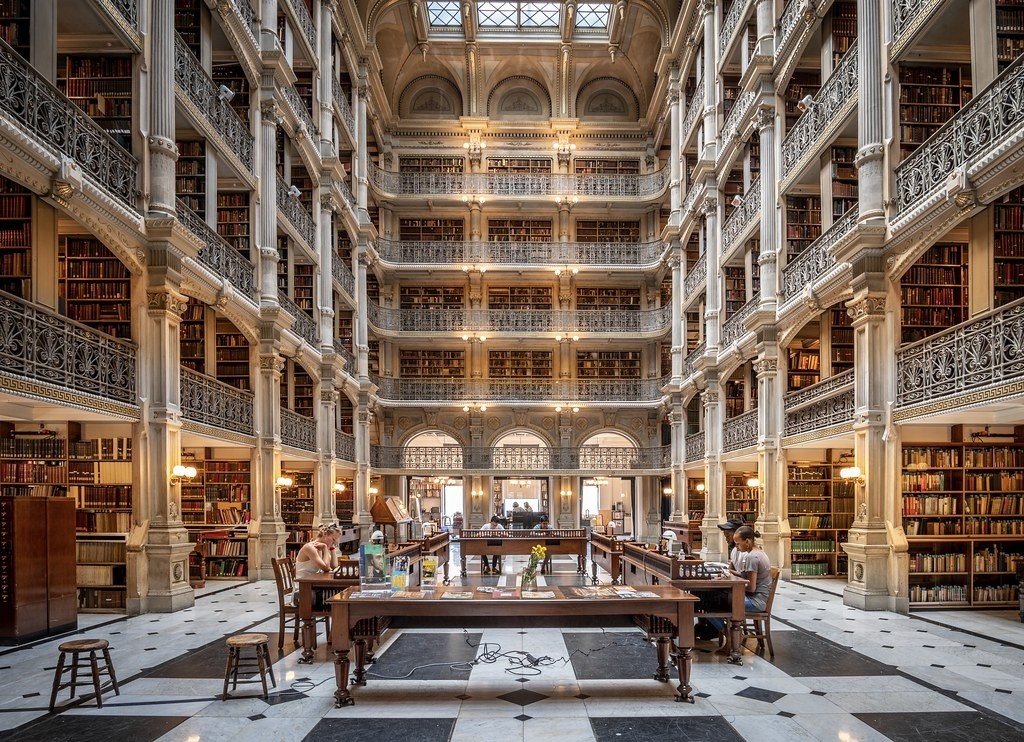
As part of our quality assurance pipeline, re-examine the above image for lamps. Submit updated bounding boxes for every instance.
[462,265,485,273]
[554,265,579,276]
[463,139,575,150]
[463,402,579,413]
[555,331,581,342]
[460,332,486,342]
[167,465,866,500]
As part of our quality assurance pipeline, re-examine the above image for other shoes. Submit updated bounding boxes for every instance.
[492,568,499,573]
[693,624,720,640]
[484,568,490,573]
[541,568,545,574]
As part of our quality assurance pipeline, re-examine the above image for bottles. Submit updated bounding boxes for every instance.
[678,548,685,560]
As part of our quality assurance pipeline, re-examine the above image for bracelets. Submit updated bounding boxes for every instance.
[330,547,335,550]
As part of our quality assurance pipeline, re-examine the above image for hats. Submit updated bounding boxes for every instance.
[717,519,745,531]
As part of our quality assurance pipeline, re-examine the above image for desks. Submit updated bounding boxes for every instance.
[622,543,749,664]
[590,533,635,585]
[326,584,700,707]
[409,529,587,575]
[299,543,422,663]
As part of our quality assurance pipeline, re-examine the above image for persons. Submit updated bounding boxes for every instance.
[530,515,554,573]
[694,519,748,641]
[524,502,533,512]
[513,502,526,512]
[291,524,342,606]
[482,516,504,572]
[706,525,772,655]
[366,554,384,577]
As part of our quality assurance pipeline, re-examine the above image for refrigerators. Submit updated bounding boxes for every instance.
[613,512,624,534]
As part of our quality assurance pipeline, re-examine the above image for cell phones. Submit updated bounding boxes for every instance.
[729,561,736,572]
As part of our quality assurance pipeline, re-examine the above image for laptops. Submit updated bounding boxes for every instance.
[681,561,722,573]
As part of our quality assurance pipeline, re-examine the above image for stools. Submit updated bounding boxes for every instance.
[222,634,277,699]
[50,638,119,709]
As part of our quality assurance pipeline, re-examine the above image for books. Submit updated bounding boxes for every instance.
[281,473,314,563]
[336,476,354,528]
[348,545,555,600]
[181,452,251,577]
[0,0,1024,448]
[788,467,855,576]
[328,565,357,574]
[688,478,705,520]
[0,437,133,629]
[900,447,1024,602]
[726,477,759,522]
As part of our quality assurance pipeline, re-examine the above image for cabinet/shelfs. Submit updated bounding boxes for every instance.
[0,1,379,640]
[400,157,644,400]
[688,0,1023,605]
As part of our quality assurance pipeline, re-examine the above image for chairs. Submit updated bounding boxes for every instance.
[271,558,330,648]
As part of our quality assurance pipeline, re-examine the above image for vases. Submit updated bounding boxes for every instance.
[523,568,536,592]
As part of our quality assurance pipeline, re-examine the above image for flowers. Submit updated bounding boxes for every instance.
[520,546,547,567]
